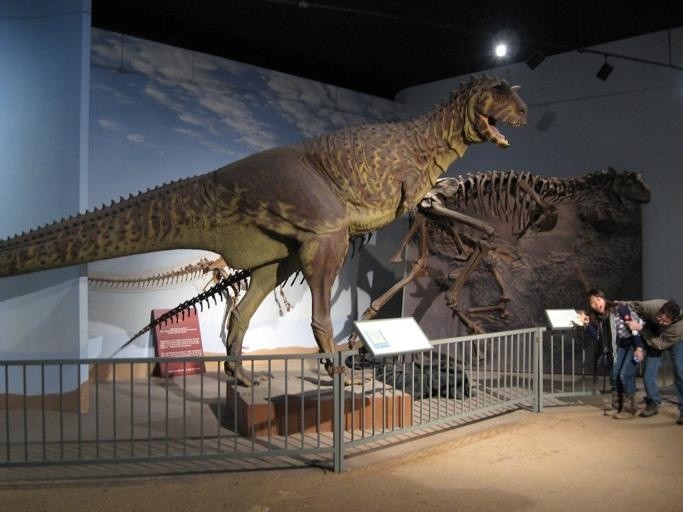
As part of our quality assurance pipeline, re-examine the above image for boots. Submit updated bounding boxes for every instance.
[603,391,622,416]
[640,397,658,417]
[615,393,636,419]
[677,406,683,423]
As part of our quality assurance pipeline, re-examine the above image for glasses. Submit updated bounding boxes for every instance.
[656,312,677,326]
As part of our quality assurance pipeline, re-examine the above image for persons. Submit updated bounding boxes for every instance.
[613,297,683,425]
[577,290,646,420]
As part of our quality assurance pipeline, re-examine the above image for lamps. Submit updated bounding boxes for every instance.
[527,45,546,70]
[594,55,613,81]
